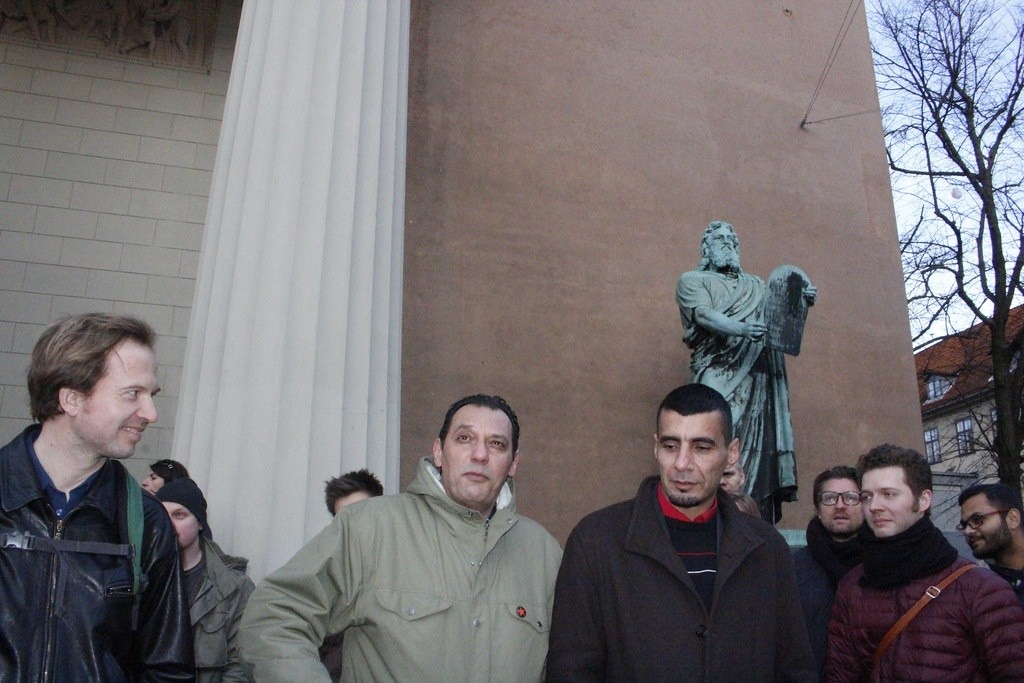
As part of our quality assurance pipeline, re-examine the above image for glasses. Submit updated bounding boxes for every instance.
[815,490,861,505]
[955,509,1009,537]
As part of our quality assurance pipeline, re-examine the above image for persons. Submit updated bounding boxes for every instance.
[677,222,817,525]
[236,394,564,683]
[539,383,816,683]
[0,312,255,683]
[792,443,1023,683]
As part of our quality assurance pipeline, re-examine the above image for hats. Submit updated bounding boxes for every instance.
[153,476,208,531]
